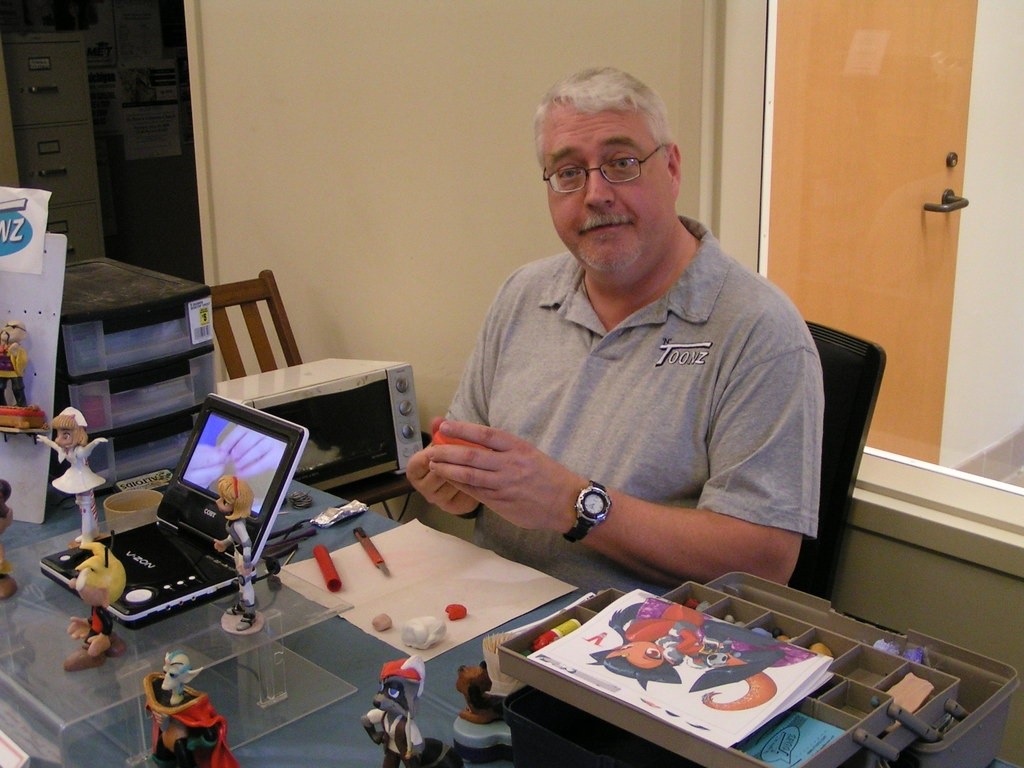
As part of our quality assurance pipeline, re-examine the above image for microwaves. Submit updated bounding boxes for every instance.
[192,358,424,493]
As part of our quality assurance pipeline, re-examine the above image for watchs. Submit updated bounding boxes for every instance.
[562,480,612,544]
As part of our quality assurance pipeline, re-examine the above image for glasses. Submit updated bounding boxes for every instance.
[542,141,662,194]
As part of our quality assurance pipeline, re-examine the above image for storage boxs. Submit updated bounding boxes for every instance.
[54,346,218,434]
[55,258,215,378]
[50,402,206,504]
[0,0,182,162]
[496,570,1020,768]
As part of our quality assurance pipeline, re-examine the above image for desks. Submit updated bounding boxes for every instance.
[0,480,1024,768]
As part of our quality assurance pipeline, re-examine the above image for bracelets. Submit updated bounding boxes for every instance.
[456,502,484,520]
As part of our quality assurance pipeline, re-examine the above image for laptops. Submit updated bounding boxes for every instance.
[41,392,310,631]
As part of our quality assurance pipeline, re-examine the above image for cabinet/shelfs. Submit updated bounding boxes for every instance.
[3,30,106,260]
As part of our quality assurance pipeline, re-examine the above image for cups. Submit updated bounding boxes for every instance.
[104,488,163,536]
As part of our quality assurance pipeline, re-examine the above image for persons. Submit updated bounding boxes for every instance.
[0,320,29,408]
[37,406,109,543]
[214,474,256,632]
[405,65,827,586]
[183,425,286,491]
[360,656,425,768]
[0,478,18,600]
[64,530,127,673]
[143,650,242,768]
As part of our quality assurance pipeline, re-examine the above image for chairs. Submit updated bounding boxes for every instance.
[785,319,888,611]
[209,267,436,522]
